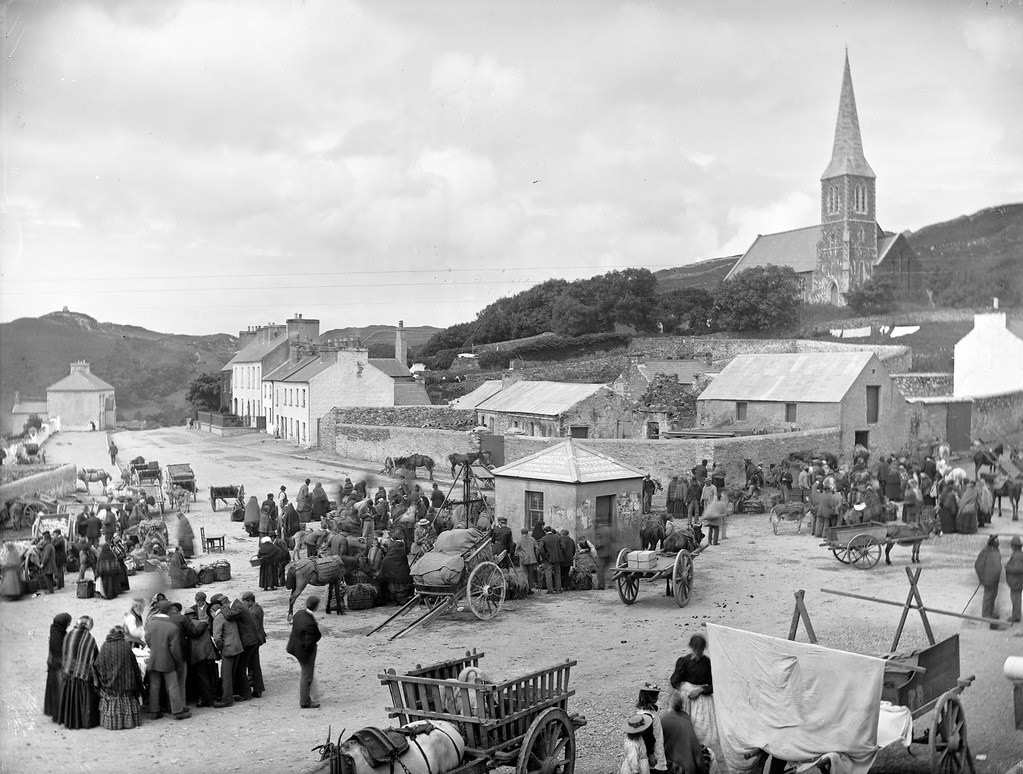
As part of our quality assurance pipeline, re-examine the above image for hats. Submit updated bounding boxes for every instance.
[185,609,196,617]
[623,713,653,733]
[242,592,252,600]
[196,592,207,599]
[638,681,661,692]
[206,600,222,618]
[987,534,998,545]
[159,600,171,612]
[418,519,430,525]
[167,602,182,612]
[500,518,507,523]
[218,596,228,601]
[1010,536,1022,546]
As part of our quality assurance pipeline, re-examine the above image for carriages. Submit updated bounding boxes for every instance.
[819,511,944,570]
[611,521,710,607]
[165,463,198,513]
[307,646,589,774]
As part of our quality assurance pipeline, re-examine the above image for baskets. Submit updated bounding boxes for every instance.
[250,556,261,567]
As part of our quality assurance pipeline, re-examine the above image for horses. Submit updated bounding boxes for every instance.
[743,459,764,490]
[304,718,465,774]
[16,445,46,465]
[285,551,379,623]
[394,453,434,481]
[641,512,673,550]
[974,444,1003,481]
[449,451,484,478]
[980,473,1021,521]
[78,468,112,495]
[662,524,706,596]
[769,502,813,535]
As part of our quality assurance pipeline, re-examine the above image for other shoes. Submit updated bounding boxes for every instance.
[252,691,262,698]
[301,701,320,709]
[264,588,268,591]
[235,695,251,701]
[214,700,232,708]
[1008,618,1019,623]
[272,588,277,591]
[152,712,163,719]
[175,711,192,719]
[547,589,561,594]
[44,590,53,594]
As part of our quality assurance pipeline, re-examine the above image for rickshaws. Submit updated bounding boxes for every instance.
[743,632,978,774]
[207,484,246,512]
[123,461,163,489]
[367,533,508,642]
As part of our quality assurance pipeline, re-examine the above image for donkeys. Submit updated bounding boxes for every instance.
[884,517,943,565]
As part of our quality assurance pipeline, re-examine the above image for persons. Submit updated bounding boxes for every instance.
[0,495,199,599]
[246,476,604,607]
[644,473,655,514]
[618,714,654,774]
[44,592,267,730]
[665,443,1023,539]
[975,534,1002,618]
[635,683,669,774]
[107,442,118,465]
[287,596,323,709]
[670,633,717,746]
[231,496,246,521]
[1004,535,1023,622]
[190,419,194,429]
[175,512,195,559]
[91,422,95,430]
[661,693,721,774]
